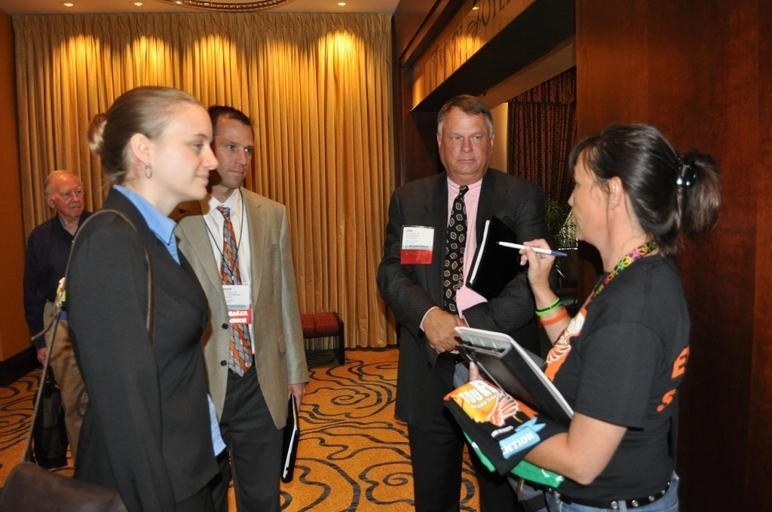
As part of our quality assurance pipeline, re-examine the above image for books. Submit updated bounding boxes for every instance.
[451,325,575,420]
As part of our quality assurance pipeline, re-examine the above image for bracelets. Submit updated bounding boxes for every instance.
[534,296,562,318]
[534,298,562,313]
[537,306,568,326]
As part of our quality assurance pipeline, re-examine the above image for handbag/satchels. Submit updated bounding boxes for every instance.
[33,366,69,467]
[0,462,126,512]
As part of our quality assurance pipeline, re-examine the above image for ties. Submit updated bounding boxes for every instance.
[217,206,252,377]
[444,185,468,314]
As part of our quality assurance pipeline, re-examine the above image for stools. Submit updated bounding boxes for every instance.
[303,312,345,366]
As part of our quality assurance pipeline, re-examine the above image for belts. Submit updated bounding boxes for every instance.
[544,482,671,509]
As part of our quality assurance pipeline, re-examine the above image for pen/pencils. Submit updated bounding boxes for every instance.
[495,241,568,258]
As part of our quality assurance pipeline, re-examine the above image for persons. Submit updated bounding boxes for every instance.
[21,168,94,470]
[64,85,221,510]
[488,390,522,426]
[168,105,311,509]
[466,122,721,511]
[375,95,554,509]
